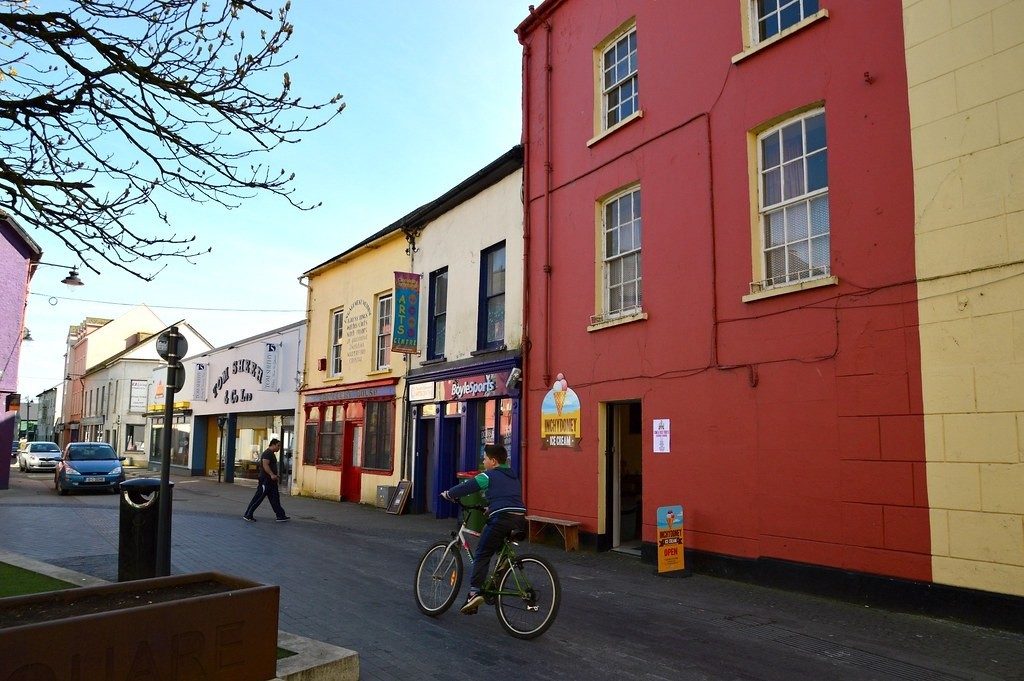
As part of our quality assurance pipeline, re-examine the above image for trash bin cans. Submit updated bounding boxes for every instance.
[456,471,489,533]
[117,477,172,582]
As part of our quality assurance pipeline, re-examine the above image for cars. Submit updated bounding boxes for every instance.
[55,442,126,496]
[12,441,20,457]
[16,441,63,473]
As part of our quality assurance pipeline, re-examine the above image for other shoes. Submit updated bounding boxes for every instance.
[243,516,256,522]
[276,517,290,521]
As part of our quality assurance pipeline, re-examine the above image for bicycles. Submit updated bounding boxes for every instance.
[413,493,562,639]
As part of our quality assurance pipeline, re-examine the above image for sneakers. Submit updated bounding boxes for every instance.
[459,591,484,612]
[493,554,511,574]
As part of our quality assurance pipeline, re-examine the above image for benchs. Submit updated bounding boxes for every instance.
[525,515,582,552]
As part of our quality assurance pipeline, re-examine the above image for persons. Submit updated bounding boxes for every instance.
[243,438,291,522]
[441,445,527,612]
[15,434,27,465]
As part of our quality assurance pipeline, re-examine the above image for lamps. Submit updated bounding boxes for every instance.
[64,373,83,382]
[27,262,85,287]
[23,326,34,341]
